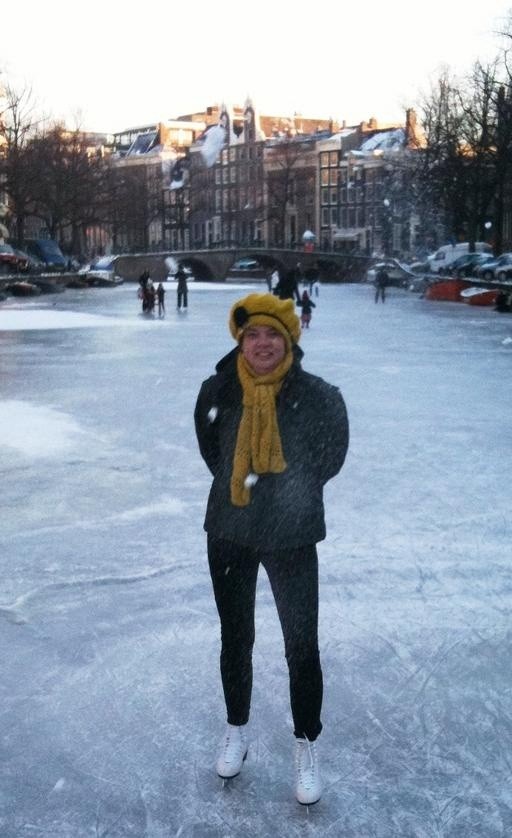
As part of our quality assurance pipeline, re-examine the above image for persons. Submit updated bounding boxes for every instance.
[373,266,389,304]
[265,267,277,292]
[192,288,352,804]
[137,266,167,316]
[295,290,316,329]
[274,269,302,306]
[84,229,417,265]
[270,269,280,293]
[172,263,191,310]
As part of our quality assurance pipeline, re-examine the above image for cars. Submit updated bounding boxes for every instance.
[366,242,511,282]
[0,237,65,274]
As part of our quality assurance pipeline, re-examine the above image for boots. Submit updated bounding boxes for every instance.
[293,737,323,808]
[214,722,249,781]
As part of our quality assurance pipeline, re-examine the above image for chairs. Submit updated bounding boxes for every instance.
[423,279,463,302]
[460,287,499,306]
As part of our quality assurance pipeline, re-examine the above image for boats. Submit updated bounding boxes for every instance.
[85,270,124,286]
[12,279,65,295]
[424,280,498,305]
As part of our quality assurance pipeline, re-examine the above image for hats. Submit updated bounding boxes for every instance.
[228,292,303,350]
